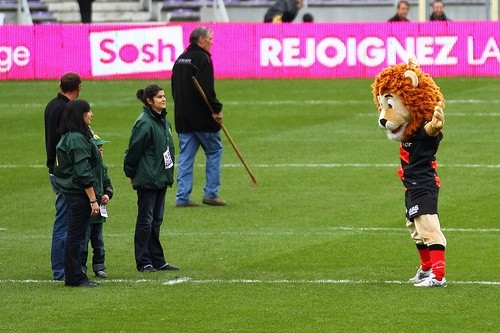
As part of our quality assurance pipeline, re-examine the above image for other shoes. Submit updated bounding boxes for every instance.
[137,267,157,272]
[95,271,109,279]
[203,198,225,205]
[177,202,199,207]
[88,282,100,285]
[78,283,97,287]
[161,265,179,270]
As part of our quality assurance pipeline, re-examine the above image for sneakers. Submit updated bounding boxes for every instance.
[410,268,432,282]
[414,273,448,287]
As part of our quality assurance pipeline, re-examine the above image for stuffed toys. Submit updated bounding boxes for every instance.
[371,57,448,287]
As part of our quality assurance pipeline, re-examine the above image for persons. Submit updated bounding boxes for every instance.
[51,100,103,289]
[82,135,114,279]
[44,73,81,280]
[263,0,303,23]
[427,0,452,21]
[171,26,226,208]
[123,85,180,273]
[302,13,314,22]
[388,1,410,21]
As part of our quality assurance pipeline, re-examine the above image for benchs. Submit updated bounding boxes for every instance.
[18,0,171,23]
